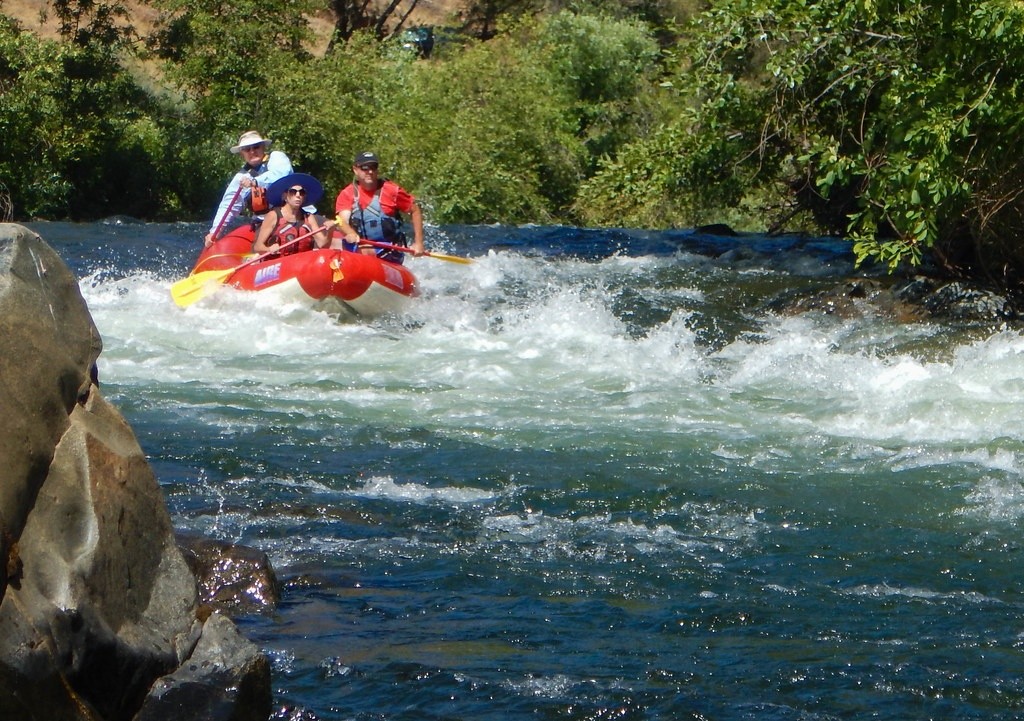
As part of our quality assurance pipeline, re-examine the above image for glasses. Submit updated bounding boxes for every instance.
[242,143,260,152]
[288,188,306,196]
[358,164,377,171]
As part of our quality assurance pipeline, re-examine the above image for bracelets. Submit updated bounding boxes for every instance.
[252,178,258,186]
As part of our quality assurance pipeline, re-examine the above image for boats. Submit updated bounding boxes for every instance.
[184,219,423,326]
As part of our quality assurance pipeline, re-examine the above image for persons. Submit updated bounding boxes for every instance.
[204,131,424,264]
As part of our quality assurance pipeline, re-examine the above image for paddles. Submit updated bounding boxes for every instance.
[359,238,474,264]
[169,214,344,307]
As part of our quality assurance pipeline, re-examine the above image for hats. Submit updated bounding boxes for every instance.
[230,131,272,153]
[353,151,378,167]
[267,173,322,207]
